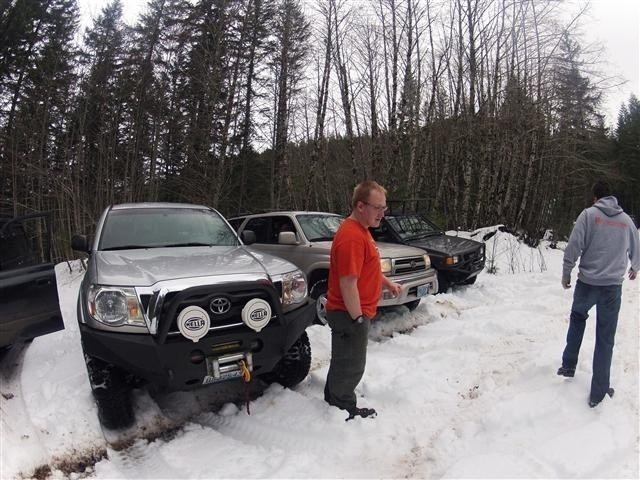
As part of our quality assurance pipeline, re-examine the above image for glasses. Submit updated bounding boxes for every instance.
[359,200,388,212]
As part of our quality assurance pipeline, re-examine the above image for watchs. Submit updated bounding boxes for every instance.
[352,314,365,327]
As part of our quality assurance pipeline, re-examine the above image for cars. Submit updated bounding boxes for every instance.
[0,209,65,379]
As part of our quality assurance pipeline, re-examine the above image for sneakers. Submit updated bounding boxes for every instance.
[590,388,614,407]
[340,406,377,421]
[557,368,575,377]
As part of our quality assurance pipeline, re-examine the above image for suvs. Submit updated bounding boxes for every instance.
[225,211,440,326]
[77,201,317,430]
[346,213,485,295]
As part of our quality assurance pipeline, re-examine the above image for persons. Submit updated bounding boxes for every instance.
[321,180,403,421]
[557,180,639,408]
